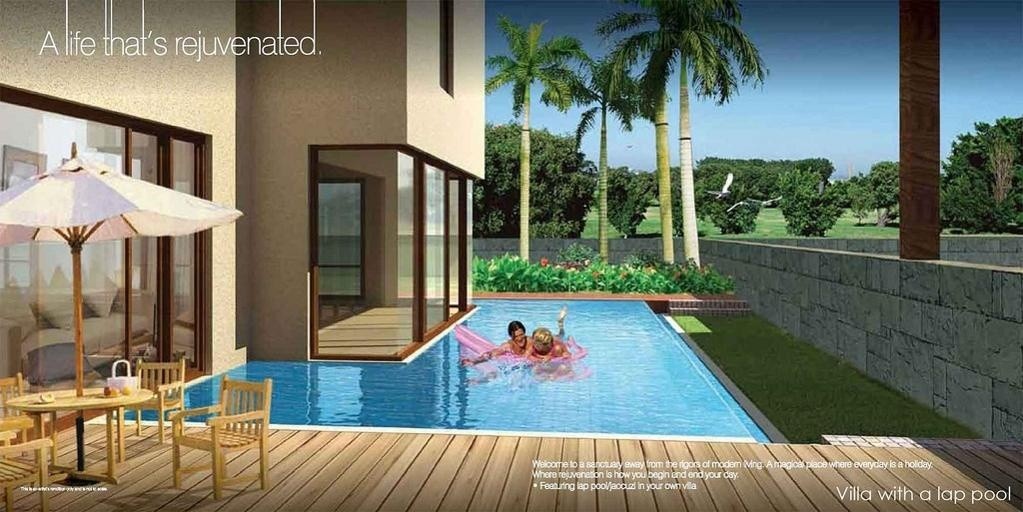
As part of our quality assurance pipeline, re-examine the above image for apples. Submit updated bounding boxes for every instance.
[104,386,111,395]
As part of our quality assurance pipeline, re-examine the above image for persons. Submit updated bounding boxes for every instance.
[459,303,569,368]
[467,368,580,390]
[526,326,565,362]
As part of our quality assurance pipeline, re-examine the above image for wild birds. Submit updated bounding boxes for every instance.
[707,173,733,199]
[726,201,750,213]
[745,195,784,206]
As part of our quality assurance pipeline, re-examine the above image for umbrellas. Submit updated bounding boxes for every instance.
[2,138,245,470]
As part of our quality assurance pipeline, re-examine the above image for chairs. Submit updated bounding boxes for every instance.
[0,357,270,500]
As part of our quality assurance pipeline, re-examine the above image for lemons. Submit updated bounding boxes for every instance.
[122,385,131,395]
[111,388,119,395]
